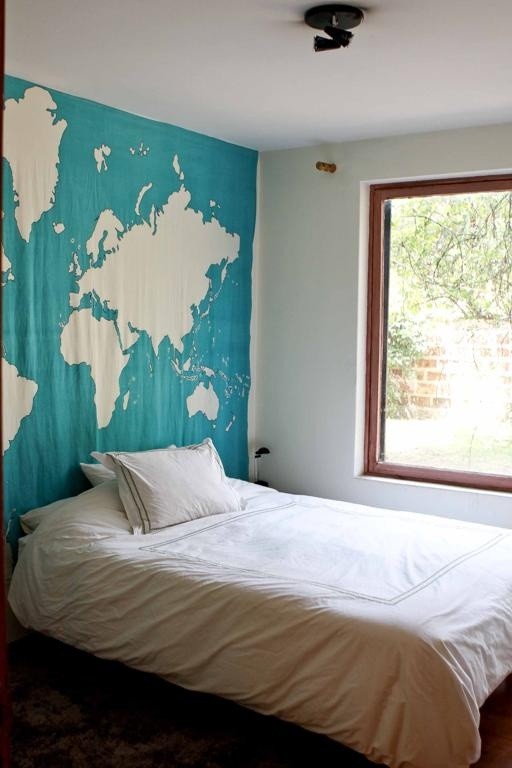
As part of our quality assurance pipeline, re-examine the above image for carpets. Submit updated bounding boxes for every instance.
[6,633,386,767]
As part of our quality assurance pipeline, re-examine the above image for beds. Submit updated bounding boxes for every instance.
[15,478,510,768]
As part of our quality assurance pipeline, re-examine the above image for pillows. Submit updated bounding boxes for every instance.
[79,436,248,533]
